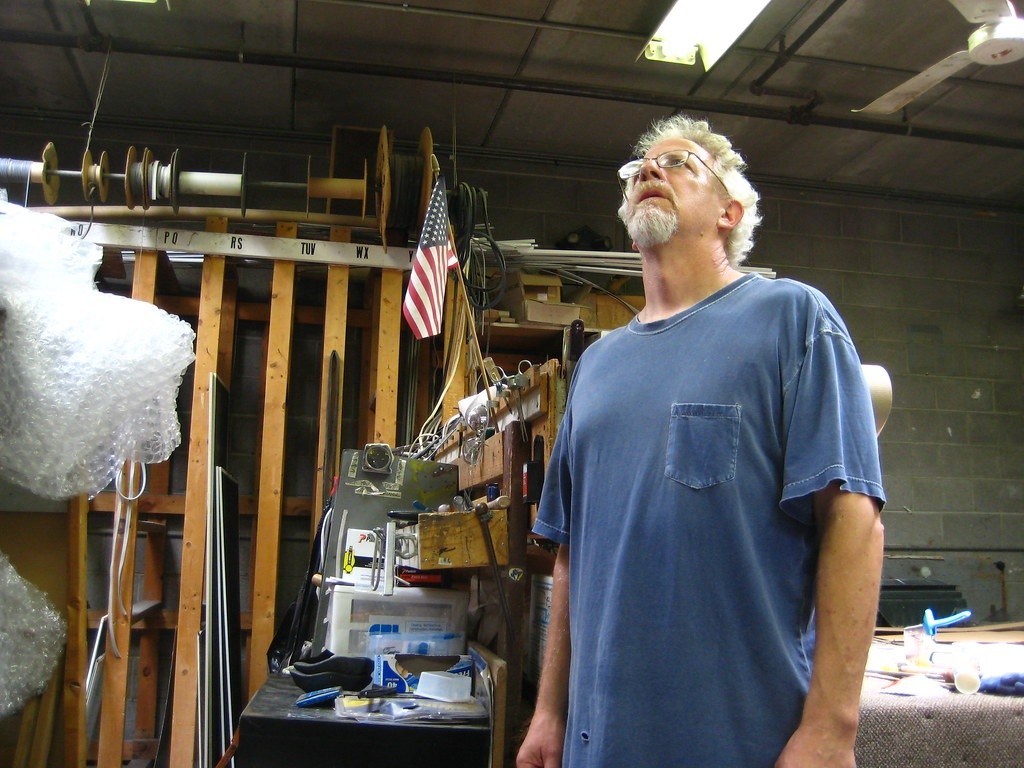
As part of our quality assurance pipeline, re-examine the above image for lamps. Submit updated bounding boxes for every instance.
[634,0,770,72]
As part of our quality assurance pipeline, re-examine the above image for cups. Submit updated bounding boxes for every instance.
[954,668,981,695]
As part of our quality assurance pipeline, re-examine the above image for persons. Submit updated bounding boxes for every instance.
[514,114,887,768]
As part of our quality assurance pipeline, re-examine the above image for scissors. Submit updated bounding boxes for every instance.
[490,360,534,442]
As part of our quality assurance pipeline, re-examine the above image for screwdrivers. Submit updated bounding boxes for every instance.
[483,357,513,414]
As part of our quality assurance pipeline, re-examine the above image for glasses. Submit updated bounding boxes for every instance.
[617,150,729,202]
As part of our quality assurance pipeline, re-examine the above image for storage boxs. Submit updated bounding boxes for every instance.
[326,587,467,655]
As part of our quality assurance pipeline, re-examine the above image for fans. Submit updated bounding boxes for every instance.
[851,0,1024,116]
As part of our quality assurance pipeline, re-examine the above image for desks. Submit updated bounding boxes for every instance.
[237,655,489,768]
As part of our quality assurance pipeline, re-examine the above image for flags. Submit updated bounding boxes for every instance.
[403,174,459,340]
